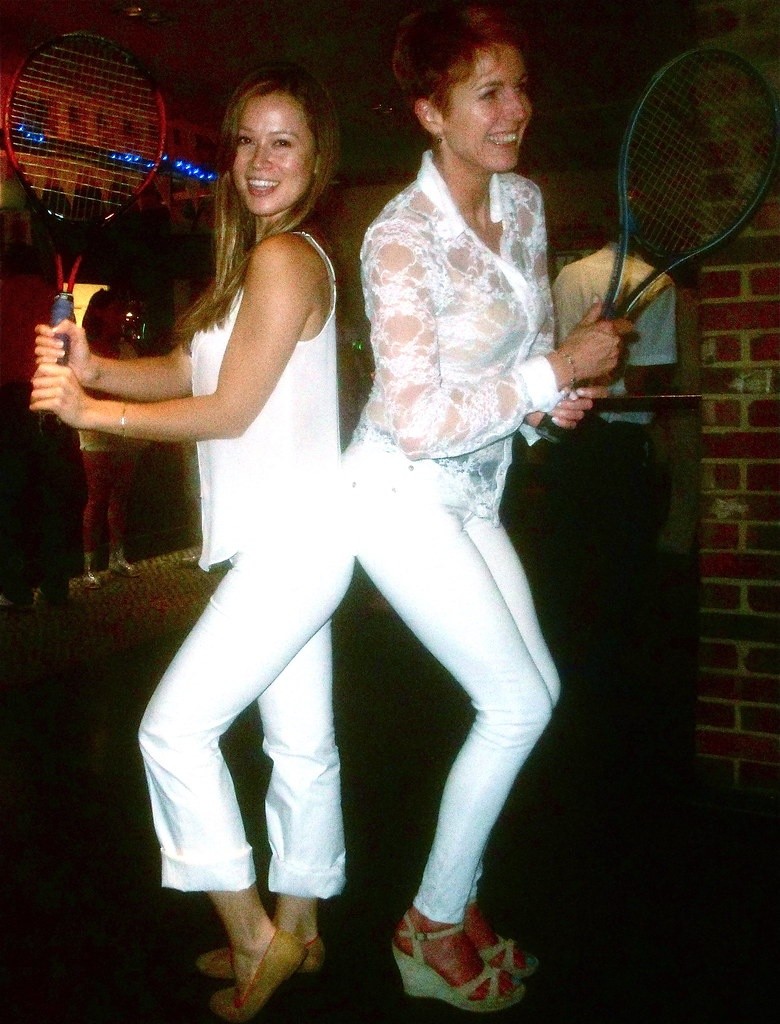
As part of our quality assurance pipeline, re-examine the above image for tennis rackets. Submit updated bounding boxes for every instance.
[535,47,780,442]
[5,32,166,435]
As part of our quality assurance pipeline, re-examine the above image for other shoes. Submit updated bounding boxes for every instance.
[211,930,306,1021]
[194,930,326,978]
[179,554,202,567]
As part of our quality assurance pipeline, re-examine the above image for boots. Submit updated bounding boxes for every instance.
[81,553,101,588]
[109,547,140,576]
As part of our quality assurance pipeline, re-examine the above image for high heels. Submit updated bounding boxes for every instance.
[393,909,526,1012]
[477,935,539,978]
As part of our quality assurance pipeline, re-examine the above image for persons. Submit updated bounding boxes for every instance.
[550,179,696,669]
[76,289,142,590]
[28,60,354,1024]
[341,1,635,1012]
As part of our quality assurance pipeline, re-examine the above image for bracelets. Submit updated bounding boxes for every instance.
[121,402,127,437]
[553,347,577,387]
